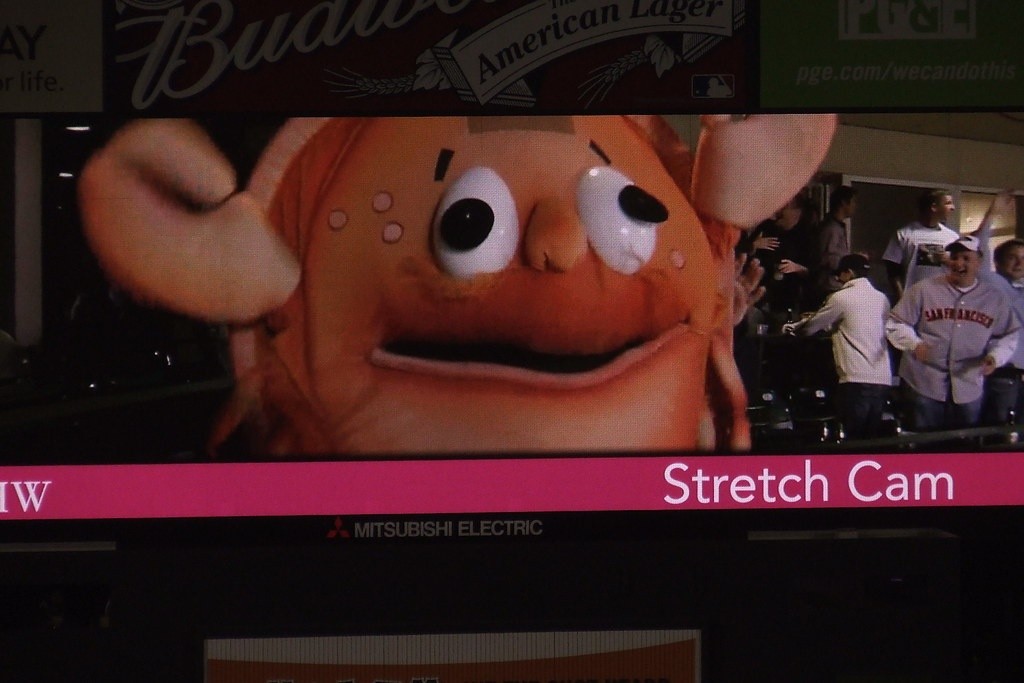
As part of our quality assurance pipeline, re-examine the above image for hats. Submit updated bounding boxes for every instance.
[945,235,983,257]
[830,254,870,274]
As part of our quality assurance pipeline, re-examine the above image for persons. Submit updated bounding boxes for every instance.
[734,187,1024,446]
